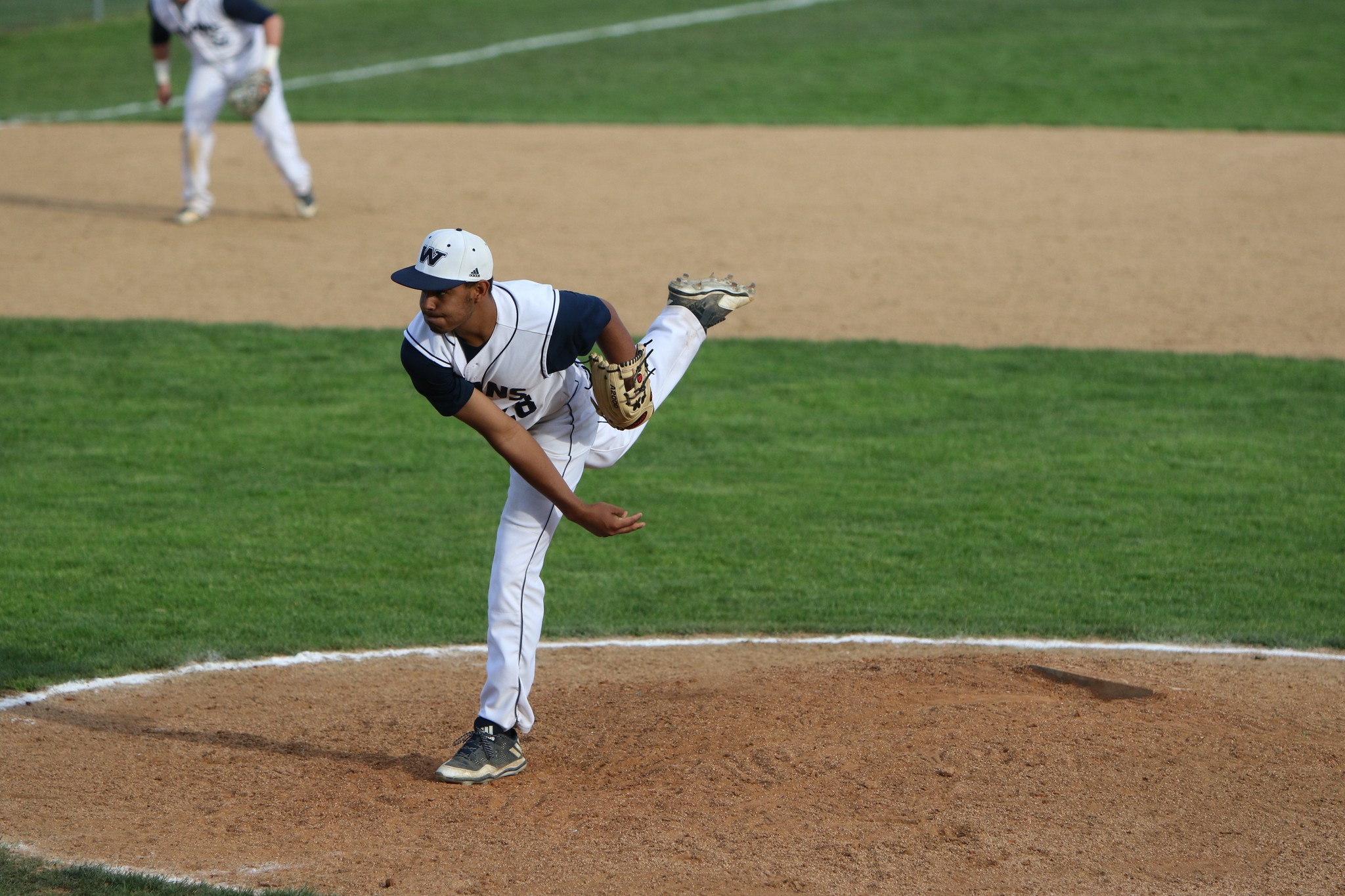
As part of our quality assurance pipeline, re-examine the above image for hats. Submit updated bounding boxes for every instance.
[390,229,494,292]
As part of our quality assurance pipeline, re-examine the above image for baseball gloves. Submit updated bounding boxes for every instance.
[225,73,274,119]
[589,343,654,430]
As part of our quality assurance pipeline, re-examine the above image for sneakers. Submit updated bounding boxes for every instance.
[667,272,759,329]
[435,715,527,785]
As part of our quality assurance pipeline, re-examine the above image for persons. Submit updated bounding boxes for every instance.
[146,0,320,226]
[390,229,755,784]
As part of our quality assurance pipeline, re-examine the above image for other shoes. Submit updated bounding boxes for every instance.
[169,204,206,224]
[296,184,318,218]
